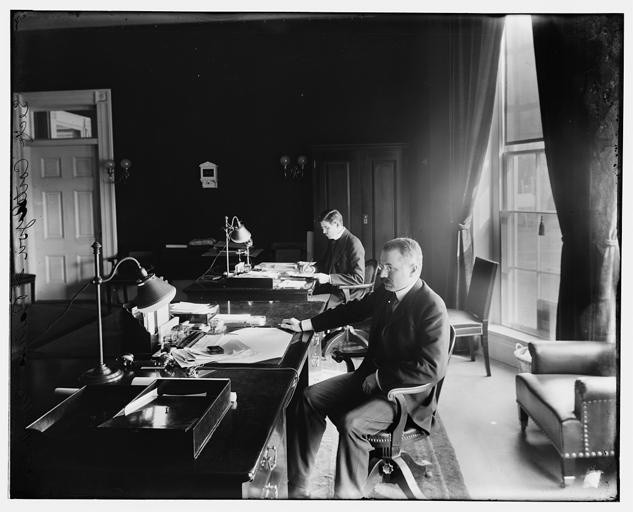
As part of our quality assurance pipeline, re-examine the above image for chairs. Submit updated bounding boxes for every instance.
[515,335,616,487]
[327,321,456,499]
[322,258,378,357]
[444,256,499,376]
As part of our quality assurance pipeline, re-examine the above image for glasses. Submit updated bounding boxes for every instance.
[377,263,391,272]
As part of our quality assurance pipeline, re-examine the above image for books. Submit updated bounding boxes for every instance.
[164,292,293,367]
[253,258,320,290]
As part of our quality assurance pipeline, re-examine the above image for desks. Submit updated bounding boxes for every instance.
[12,261,333,499]
[11,273,35,306]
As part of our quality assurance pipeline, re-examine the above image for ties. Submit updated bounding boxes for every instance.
[383,293,396,324]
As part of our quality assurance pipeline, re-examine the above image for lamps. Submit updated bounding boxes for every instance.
[102,158,132,184]
[279,155,309,182]
[221,215,251,279]
[76,241,177,387]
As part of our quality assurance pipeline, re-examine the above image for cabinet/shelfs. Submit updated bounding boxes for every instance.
[311,144,408,272]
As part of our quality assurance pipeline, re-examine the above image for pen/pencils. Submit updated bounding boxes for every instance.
[277,323,280,326]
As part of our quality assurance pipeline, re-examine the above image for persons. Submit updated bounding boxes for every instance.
[309,209,365,304]
[285,236,450,499]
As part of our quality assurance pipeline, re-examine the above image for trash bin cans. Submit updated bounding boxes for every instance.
[513,343,532,374]
